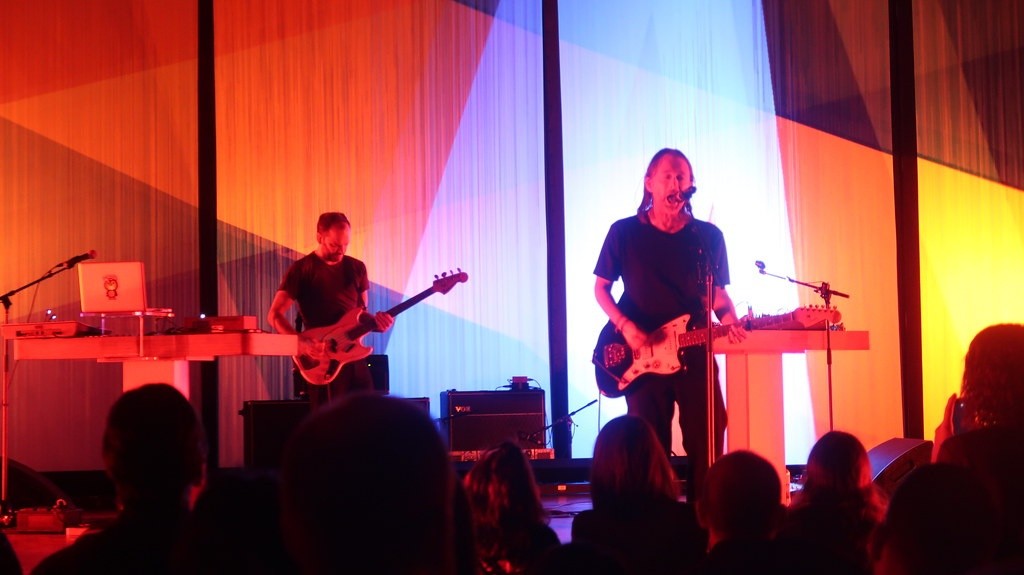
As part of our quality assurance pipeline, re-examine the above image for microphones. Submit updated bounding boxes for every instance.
[58,249,97,268]
[520,433,544,445]
[676,185,696,201]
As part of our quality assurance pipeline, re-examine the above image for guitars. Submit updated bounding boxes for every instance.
[594,306,842,398]
[294,267,468,384]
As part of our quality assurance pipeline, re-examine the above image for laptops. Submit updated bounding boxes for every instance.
[78,261,172,313]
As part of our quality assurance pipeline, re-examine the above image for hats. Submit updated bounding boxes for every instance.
[801,429,872,493]
[99,382,211,467]
[319,211,350,232]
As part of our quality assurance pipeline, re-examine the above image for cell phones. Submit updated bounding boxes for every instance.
[952,397,970,435]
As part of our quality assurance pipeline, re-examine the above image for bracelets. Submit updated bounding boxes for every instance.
[614,315,630,333]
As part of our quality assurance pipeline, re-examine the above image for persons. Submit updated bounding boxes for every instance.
[265,212,394,412]
[591,147,748,503]
[32,323,1024,575]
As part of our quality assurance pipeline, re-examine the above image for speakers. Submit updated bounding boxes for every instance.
[440,389,546,450]
[867,438,935,494]
[239,356,431,470]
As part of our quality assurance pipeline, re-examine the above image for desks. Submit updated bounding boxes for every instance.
[13,334,312,506]
[712,330,871,503]
